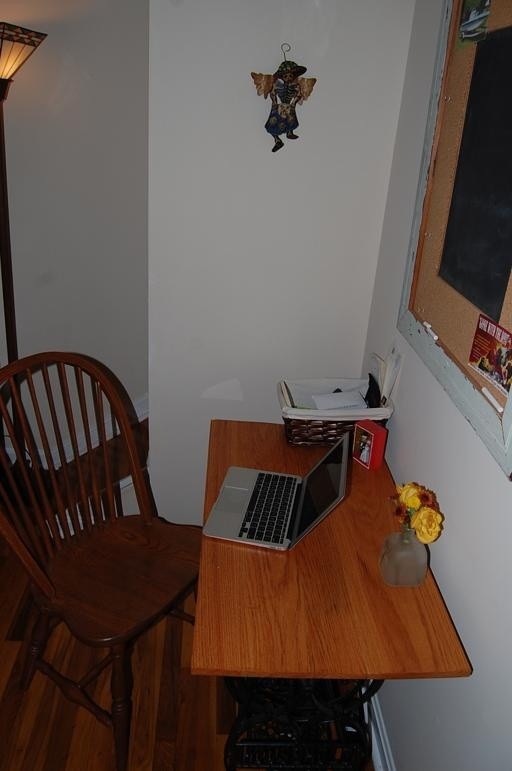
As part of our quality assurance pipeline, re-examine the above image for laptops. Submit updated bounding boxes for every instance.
[202,431,352,556]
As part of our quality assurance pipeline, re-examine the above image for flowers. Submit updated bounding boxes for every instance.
[384,480,445,544]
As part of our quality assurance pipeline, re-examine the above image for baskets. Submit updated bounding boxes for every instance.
[276,379,392,450]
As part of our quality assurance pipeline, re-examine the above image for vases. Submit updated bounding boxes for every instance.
[379,528,429,586]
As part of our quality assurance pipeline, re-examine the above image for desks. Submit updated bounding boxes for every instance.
[191,414,472,771]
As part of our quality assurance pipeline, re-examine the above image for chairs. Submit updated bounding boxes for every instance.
[0,348,201,770]
